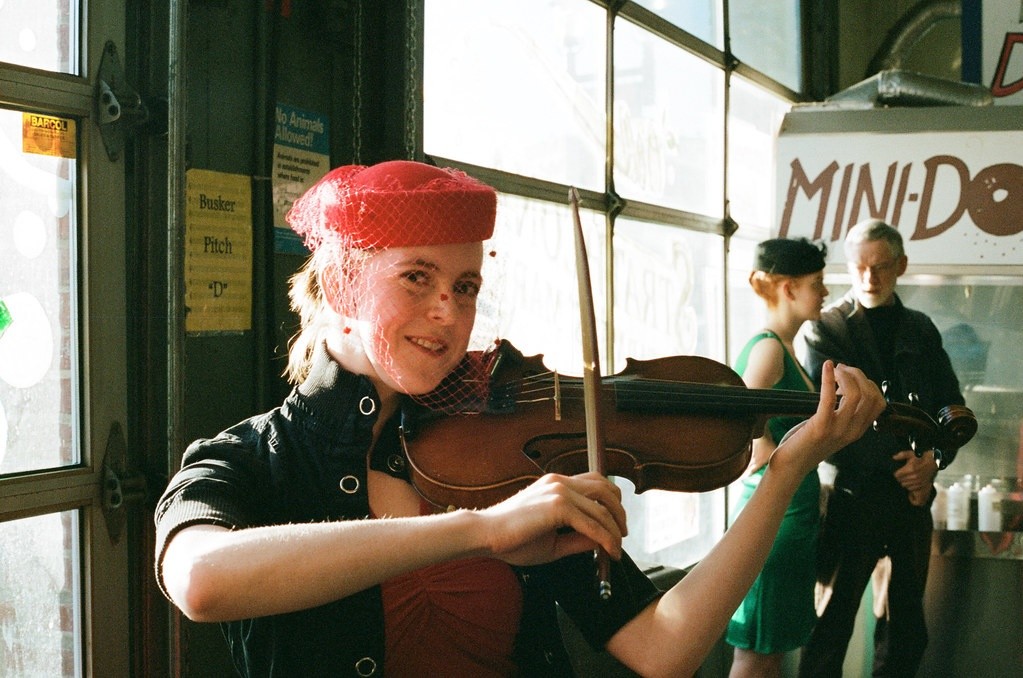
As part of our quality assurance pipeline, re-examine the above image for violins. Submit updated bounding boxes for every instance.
[394,348,979,508]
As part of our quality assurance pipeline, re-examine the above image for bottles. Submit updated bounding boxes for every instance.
[943,483,967,531]
[929,483,947,531]
[977,486,1002,532]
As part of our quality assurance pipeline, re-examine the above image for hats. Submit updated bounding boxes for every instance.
[285,160,496,247]
[754,237,828,275]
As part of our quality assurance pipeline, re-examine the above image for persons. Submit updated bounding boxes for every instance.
[791,219,966,678]
[720,236,832,678]
[152,158,886,677]
